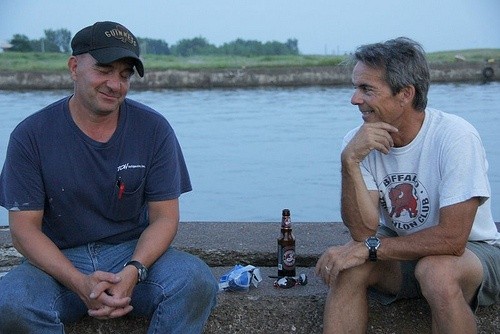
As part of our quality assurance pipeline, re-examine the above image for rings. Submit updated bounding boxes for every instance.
[325,266,331,270]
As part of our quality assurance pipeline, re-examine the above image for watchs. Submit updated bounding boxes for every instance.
[365,236,381,261]
[123,260,147,285]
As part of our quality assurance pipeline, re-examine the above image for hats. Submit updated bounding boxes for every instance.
[71,21,144,78]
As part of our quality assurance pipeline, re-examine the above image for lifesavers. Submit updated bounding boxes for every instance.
[483,67,494,78]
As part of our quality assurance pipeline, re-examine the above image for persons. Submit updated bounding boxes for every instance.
[313,35,500,334]
[0,20,220,334]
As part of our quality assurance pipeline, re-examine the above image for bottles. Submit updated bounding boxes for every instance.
[277,209,297,277]
